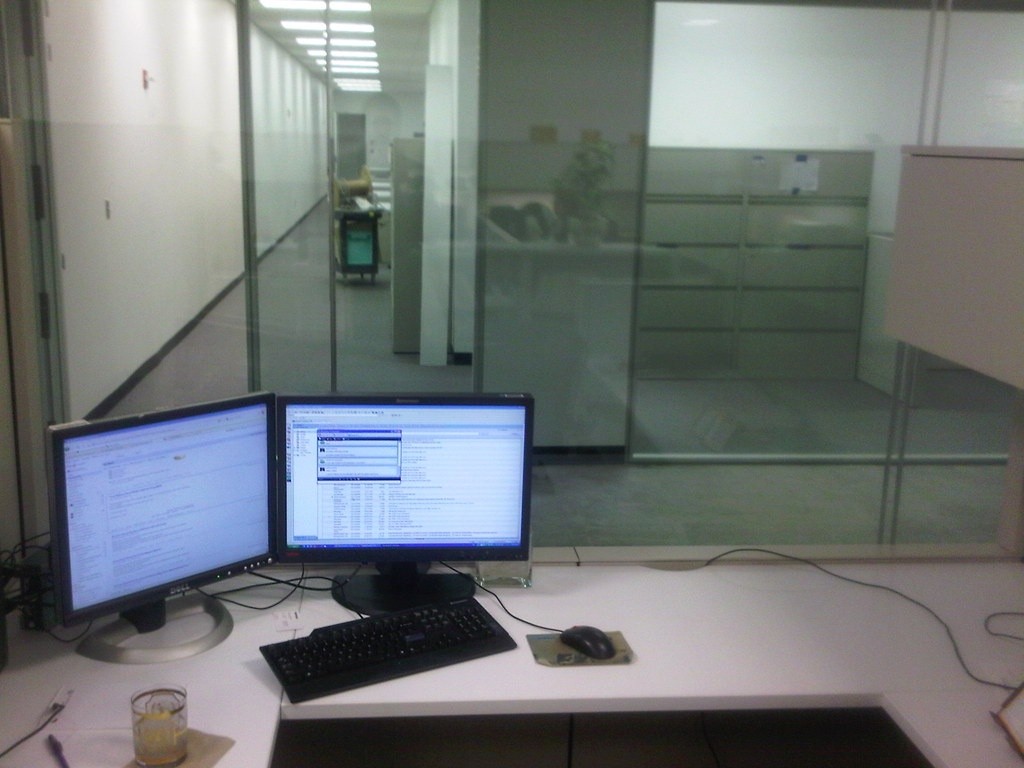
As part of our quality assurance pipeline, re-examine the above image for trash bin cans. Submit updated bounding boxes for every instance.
[332,195,381,285]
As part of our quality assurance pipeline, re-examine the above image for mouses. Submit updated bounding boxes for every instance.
[560,625,615,659]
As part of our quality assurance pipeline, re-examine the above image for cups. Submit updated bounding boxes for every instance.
[131,683,188,768]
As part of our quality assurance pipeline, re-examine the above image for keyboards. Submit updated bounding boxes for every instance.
[260,597,518,703]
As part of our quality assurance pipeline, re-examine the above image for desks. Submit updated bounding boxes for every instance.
[279,560,1024,768]
[0,570,308,768]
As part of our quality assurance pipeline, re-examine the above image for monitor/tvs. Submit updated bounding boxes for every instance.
[275,393,536,616]
[44,390,275,663]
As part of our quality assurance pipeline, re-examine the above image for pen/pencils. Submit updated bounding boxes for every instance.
[48,733,70,768]
